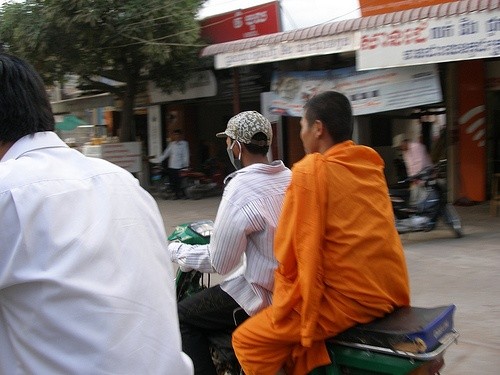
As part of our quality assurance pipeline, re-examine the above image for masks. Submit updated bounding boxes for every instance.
[227,148,241,170]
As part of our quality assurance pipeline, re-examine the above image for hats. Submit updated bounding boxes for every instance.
[216,111,272,146]
[391,134,408,148]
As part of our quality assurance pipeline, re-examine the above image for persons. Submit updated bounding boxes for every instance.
[148,130,190,200]
[0,52,195,375]
[168,110,292,375]
[231,91,410,375]
[383,132,434,219]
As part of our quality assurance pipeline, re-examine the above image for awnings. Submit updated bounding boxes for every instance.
[202,1,500,57]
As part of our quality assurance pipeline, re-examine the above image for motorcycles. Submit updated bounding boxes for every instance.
[179,166,228,200]
[166,218,462,372]
[388,156,468,238]
